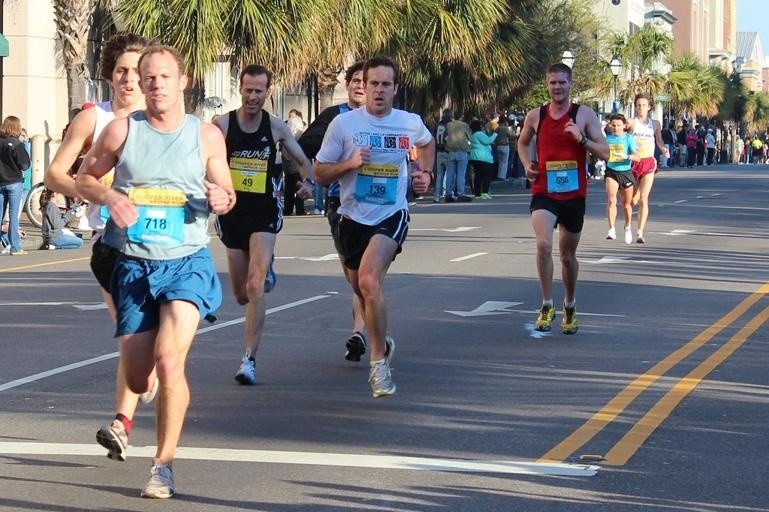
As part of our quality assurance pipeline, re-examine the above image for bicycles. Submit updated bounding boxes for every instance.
[27,175,89,231]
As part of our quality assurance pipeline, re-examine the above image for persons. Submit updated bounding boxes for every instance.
[595,114,640,244]
[312,56,435,397]
[586,112,769,180]
[0,103,98,255]
[406,108,536,206]
[297,62,395,366]
[212,109,327,216]
[624,93,670,244]
[517,63,610,335]
[44,35,158,461]
[75,44,236,499]
[211,65,316,386]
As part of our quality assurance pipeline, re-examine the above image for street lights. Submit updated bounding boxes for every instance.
[609,60,623,114]
[562,51,574,71]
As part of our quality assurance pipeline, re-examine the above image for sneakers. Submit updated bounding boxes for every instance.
[369,336,396,398]
[535,303,579,334]
[235,361,255,384]
[445,192,491,202]
[264,266,275,292]
[345,332,365,361]
[607,226,644,244]
[96,419,127,461]
[140,464,174,498]
